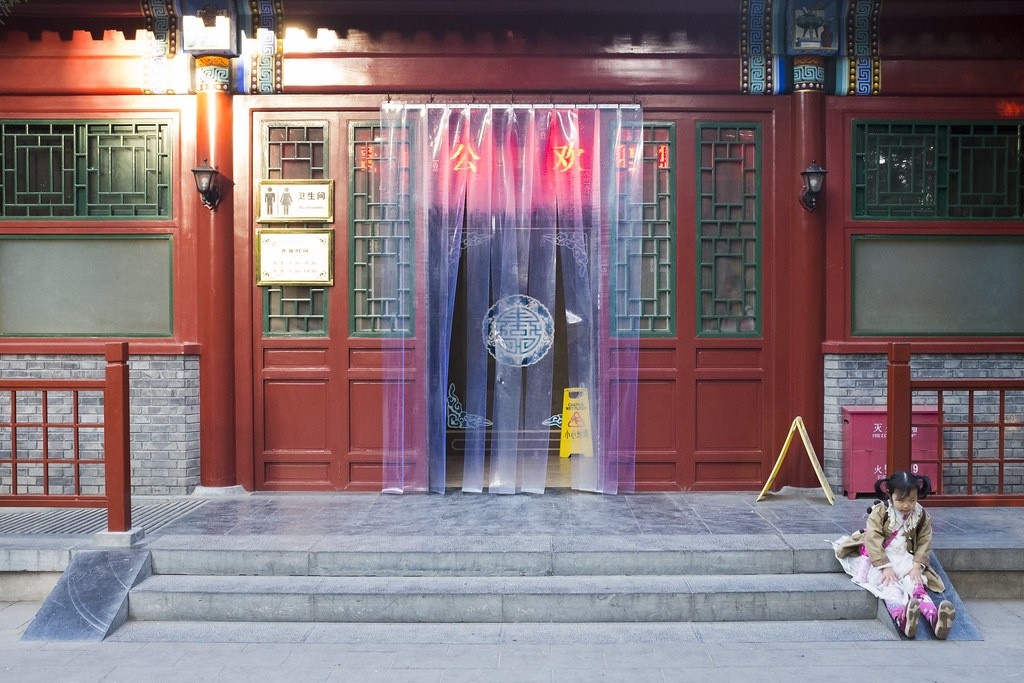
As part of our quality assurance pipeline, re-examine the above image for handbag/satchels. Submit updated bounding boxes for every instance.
[860,543,869,557]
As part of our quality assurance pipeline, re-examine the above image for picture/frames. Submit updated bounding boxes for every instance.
[255,228,334,286]
[255,178,334,222]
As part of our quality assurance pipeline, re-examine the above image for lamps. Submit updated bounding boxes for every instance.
[191,158,221,210]
[799,159,828,211]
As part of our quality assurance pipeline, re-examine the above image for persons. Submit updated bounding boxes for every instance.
[824,471,957,641]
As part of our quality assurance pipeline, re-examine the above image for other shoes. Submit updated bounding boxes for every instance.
[896,598,920,637]
[930,600,956,639]
